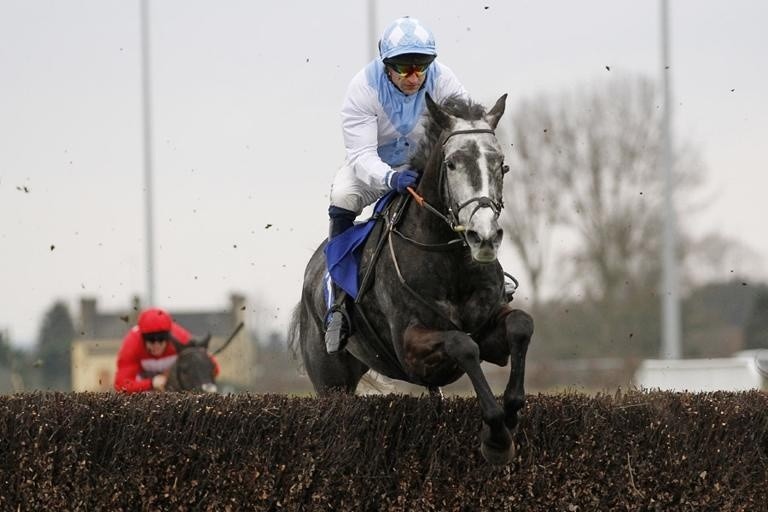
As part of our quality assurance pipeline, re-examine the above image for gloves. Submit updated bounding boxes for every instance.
[151,373,168,392]
[390,170,418,195]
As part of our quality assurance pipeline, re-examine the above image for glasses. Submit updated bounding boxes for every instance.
[145,334,166,342]
[385,62,431,75]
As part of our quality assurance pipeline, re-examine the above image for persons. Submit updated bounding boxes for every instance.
[113,307,222,394]
[324,15,469,355]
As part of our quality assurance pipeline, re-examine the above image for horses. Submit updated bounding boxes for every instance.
[285,87,535,465]
[163,332,219,394]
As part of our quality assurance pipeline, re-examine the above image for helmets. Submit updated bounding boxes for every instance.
[378,16,438,62]
[137,307,171,335]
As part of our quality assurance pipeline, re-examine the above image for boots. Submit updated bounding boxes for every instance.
[325,205,359,355]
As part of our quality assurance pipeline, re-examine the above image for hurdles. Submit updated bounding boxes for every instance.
[1,389,768,512]
